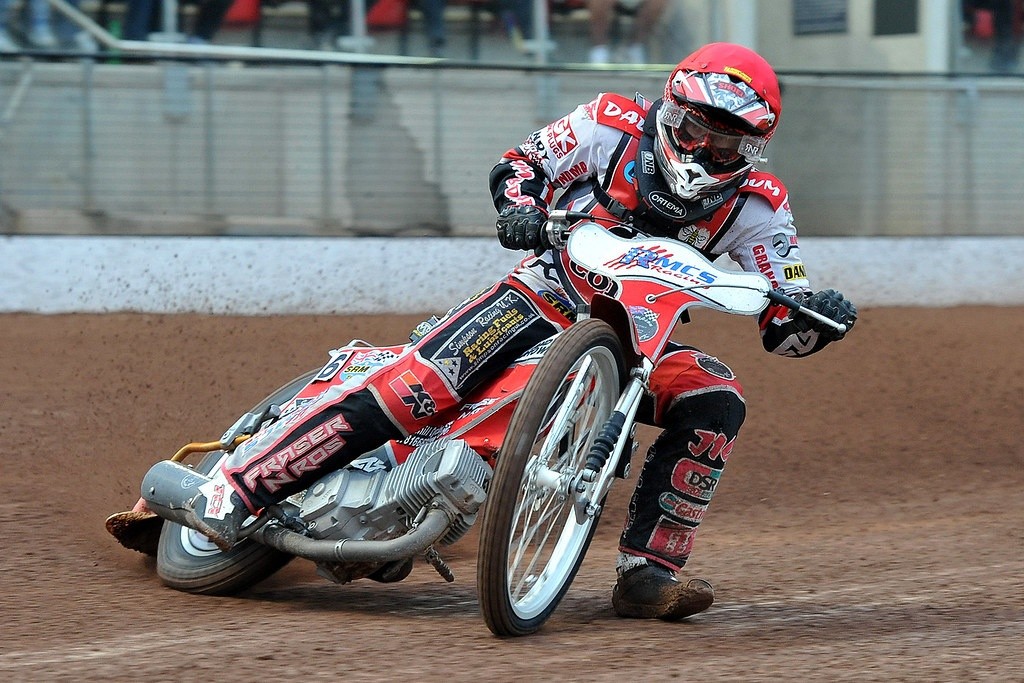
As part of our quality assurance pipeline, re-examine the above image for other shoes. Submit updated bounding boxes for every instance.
[0,29,24,54]
[26,24,57,48]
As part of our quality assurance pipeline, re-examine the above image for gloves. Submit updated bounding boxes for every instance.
[789,288,857,341]
[496,206,549,257]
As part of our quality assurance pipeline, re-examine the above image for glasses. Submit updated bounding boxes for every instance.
[684,113,743,149]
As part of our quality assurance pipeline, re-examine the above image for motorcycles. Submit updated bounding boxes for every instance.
[105,209,854,642]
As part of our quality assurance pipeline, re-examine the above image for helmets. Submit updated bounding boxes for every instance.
[654,42,782,203]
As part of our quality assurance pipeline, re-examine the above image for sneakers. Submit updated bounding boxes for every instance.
[191,484,252,552]
[612,565,714,623]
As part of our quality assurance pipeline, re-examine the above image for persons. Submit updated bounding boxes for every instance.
[0,0,669,63]
[187,42,857,619]
